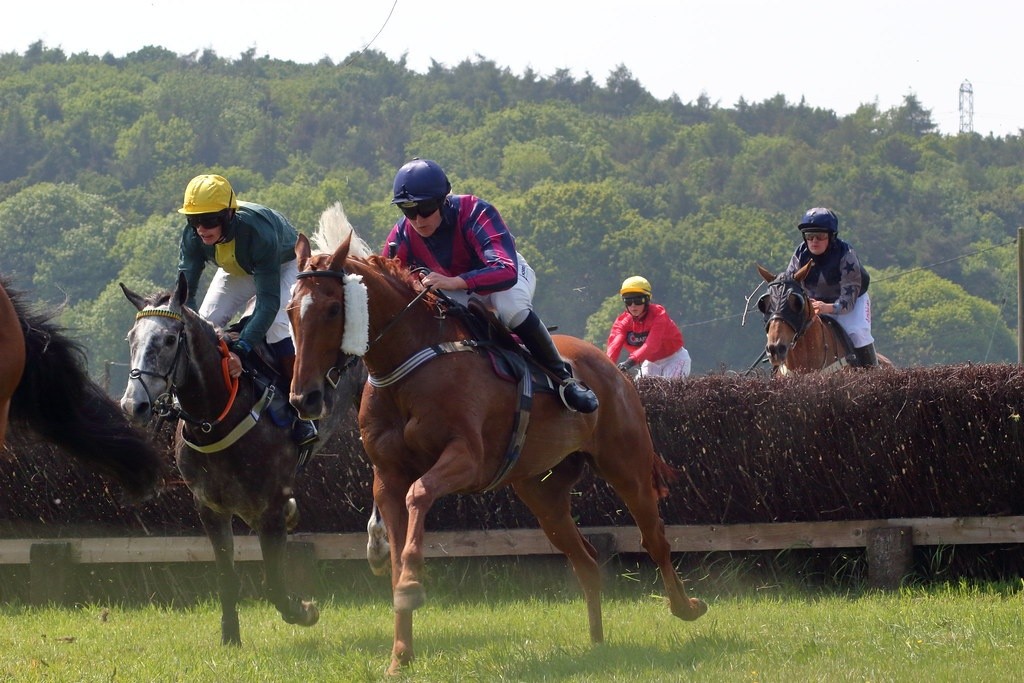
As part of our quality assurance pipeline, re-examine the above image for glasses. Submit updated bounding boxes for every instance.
[188,216,226,230]
[398,200,439,220]
[803,231,830,241]
[623,296,648,306]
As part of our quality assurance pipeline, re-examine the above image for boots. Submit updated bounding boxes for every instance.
[276,355,318,445]
[511,310,598,413]
[855,342,879,367]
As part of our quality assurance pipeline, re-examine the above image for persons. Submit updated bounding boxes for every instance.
[788,209,877,367]
[607,276,692,379]
[176,174,318,445]
[381,157,598,413]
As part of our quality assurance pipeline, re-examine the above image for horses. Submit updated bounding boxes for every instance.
[755,258,904,379]
[120,272,369,648]
[283,202,708,679]
[0,273,186,530]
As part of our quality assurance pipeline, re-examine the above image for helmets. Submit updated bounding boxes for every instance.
[177,175,239,215]
[620,275,651,296]
[799,208,837,232]
[390,156,448,203]
